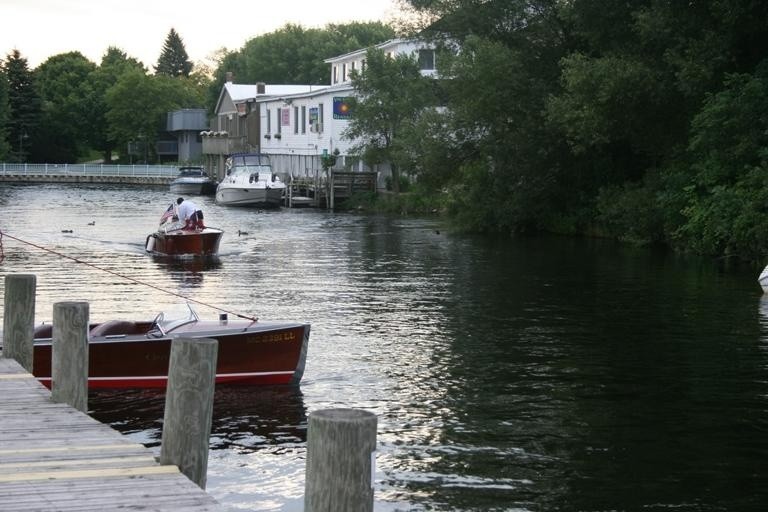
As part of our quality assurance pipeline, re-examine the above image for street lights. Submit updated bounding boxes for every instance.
[19,124,29,164]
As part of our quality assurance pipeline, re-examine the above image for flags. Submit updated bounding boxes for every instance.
[160,202,176,225]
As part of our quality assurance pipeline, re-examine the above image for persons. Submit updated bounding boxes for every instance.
[176,197,203,228]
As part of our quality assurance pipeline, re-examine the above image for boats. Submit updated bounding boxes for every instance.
[760,262,768,294]
[142,201,226,256]
[0,317,309,390]
[36,384,315,446]
[214,155,286,206]
[147,252,225,290]
[755,294,768,328]
[169,166,218,195]
